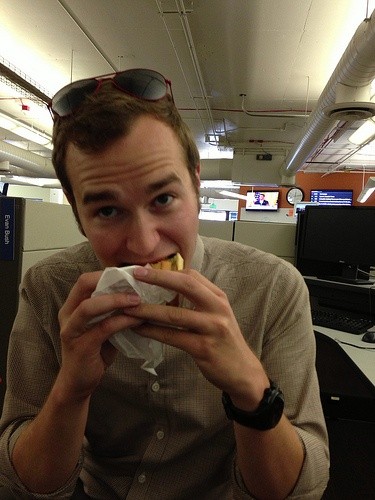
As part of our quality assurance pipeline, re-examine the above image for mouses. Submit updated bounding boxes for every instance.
[362,331,375,343]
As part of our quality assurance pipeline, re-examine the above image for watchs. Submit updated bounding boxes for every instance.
[221,379,285,430]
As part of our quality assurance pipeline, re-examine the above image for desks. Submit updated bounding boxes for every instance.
[307,321,375,393]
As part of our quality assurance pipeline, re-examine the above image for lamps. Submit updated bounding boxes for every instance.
[0,110,53,153]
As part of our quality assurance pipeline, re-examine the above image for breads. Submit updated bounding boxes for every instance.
[149,253,185,272]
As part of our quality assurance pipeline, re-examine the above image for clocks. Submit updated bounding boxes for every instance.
[286,186,305,206]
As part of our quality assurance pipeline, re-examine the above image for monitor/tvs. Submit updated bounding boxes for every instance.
[300,205,375,284]
[310,189,353,205]
[246,190,279,210]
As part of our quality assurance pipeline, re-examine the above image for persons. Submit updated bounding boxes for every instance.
[254,194,269,205]
[0,69,330,500]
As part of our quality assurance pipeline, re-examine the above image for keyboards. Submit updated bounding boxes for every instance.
[311,310,375,334]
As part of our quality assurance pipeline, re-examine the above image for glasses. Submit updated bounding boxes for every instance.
[47,69,175,124]
